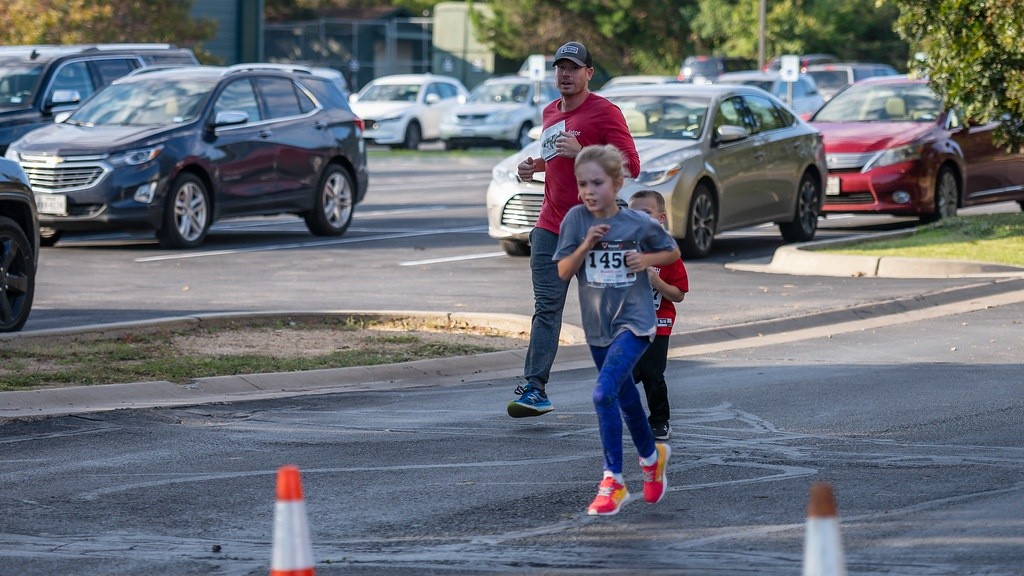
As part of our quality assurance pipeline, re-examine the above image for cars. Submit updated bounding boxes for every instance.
[486,84,827,260]
[347,54,564,150]
[0,158,40,333]
[802,74,1024,224]
[598,54,901,121]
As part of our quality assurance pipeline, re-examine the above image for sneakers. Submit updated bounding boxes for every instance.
[588,470,630,515]
[653,420,671,440]
[639,443,671,505]
[507,383,554,418]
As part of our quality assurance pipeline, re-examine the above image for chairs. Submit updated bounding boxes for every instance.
[622,109,656,138]
[884,96,913,120]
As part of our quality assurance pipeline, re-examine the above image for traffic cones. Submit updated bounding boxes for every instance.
[269,466,319,576]
[802,482,851,576]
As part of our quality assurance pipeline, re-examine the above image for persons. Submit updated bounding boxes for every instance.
[626,190,688,440]
[506,40,641,418]
[552,144,681,515]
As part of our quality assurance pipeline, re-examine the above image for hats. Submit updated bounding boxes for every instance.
[552,41,591,68]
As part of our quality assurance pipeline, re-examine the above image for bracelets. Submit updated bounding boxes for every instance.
[581,144,583,149]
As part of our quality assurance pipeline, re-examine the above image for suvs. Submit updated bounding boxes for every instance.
[4,63,369,247]
[1,43,201,155]
